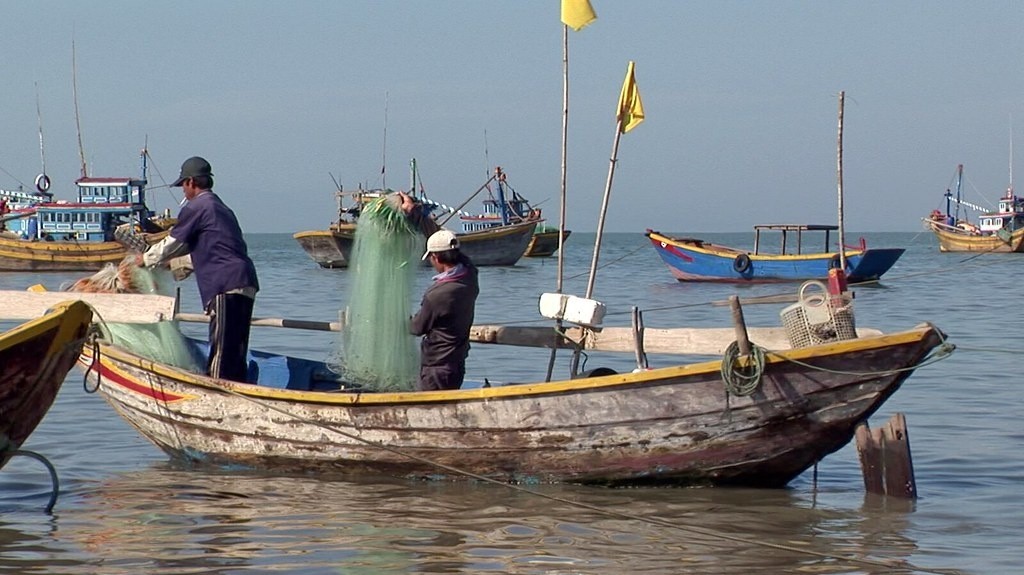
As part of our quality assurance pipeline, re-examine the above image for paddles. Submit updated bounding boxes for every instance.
[0,292,172,326]
[182,311,875,352]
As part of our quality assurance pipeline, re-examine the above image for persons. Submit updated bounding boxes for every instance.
[127,155,260,382]
[397,190,480,391]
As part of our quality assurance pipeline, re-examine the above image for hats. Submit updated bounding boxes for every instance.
[169,157,213,188]
[422,230,461,261]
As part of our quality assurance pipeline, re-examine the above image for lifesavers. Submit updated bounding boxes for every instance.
[35,173,51,192]
[733,252,750,272]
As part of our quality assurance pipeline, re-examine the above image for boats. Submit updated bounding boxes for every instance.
[1,38,181,273]
[0,295,112,471]
[26,0,956,491]
[459,130,573,258]
[924,116,1024,254]
[642,223,908,283]
[292,90,548,269]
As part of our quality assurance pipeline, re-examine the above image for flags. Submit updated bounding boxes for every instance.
[560,0,597,31]
[616,60,645,135]
